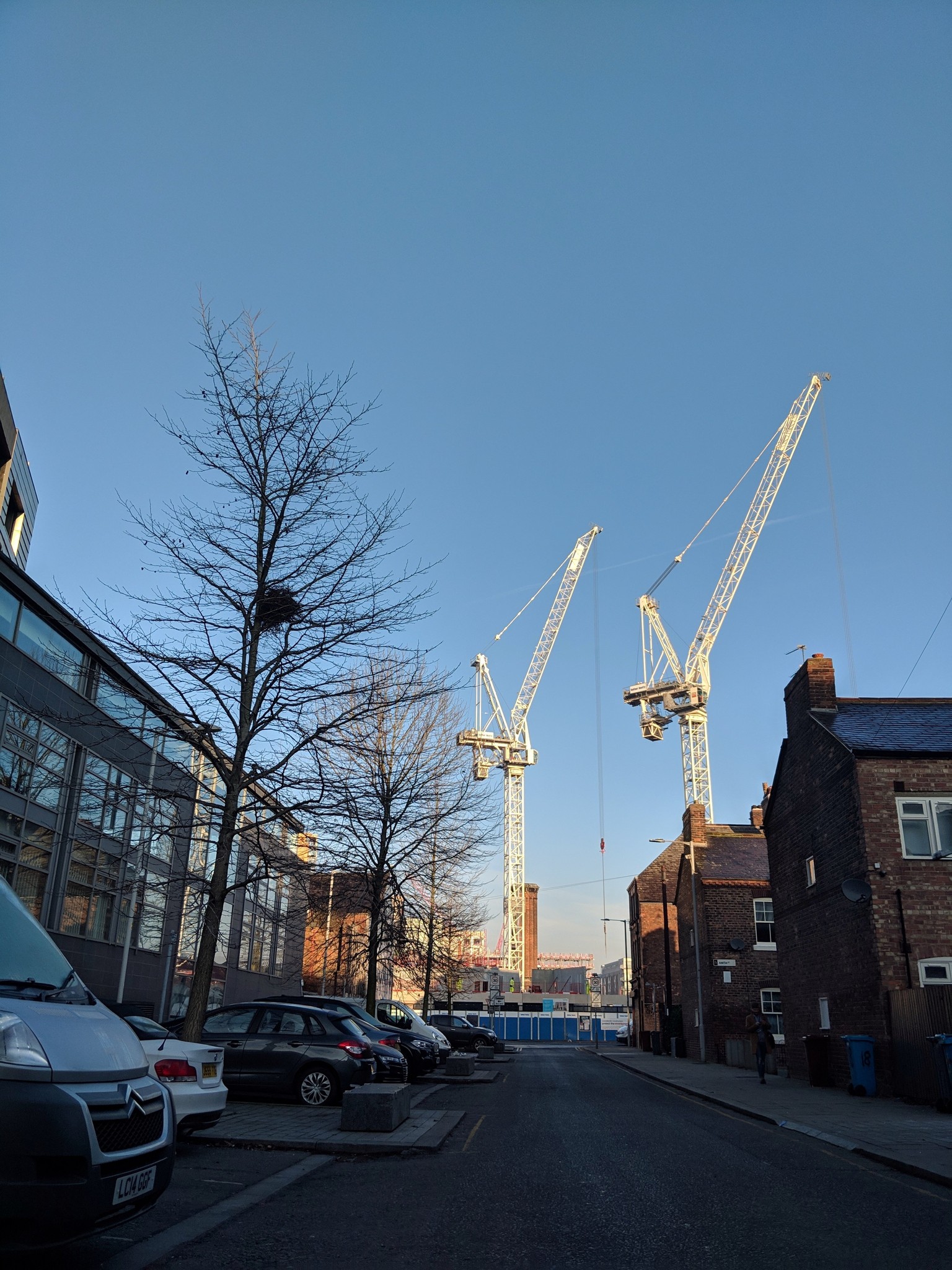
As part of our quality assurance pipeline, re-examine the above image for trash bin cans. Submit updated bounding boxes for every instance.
[803,1034,831,1088]
[641,1030,650,1052]
[840,1035,876,1096]
[650,1031,660,1055]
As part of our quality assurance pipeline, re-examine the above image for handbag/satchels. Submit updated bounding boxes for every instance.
[767,1032,776,1049]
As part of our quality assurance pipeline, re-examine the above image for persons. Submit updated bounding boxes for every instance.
[455,978,463,991]
[509,977,514,992]
[746,1003,771,1084]
[586,979,590,995]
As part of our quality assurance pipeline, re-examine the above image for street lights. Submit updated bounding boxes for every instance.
[650,838,707,1063]
[601,919,631,1047]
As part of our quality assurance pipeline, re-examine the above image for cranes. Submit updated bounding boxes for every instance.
[623,374,829,825]
[456,527,602,992]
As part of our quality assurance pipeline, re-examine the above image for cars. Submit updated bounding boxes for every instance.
[345,1014,402,1053]
[615,1021,633,1046]
[159,1002,378,1106]
[372,1041,409,1084]
[0,874,177,1270]
[252,995,441,1084]
[429,1015,498,1053]
[122,1016,228,1143]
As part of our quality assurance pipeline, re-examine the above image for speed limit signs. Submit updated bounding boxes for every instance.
[592,979,599,986]
[492,977,499,984]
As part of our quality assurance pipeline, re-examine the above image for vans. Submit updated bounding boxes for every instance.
[305,994,452,1072]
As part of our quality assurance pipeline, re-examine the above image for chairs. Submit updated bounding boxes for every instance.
[281,1021,295,1033]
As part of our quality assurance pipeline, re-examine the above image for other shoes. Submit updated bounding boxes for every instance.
[760,1078,766,1084]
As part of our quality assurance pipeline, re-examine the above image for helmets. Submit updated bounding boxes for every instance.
[512,977,514,979]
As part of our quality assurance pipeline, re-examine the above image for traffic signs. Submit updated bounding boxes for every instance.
[494,995,505,1006]
